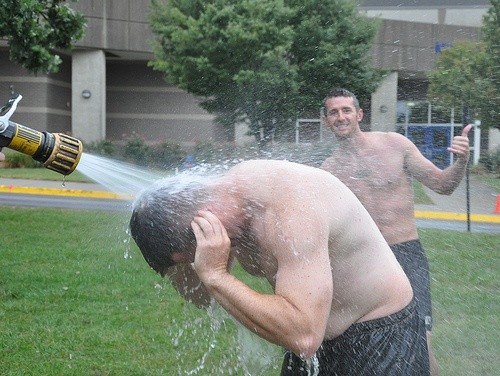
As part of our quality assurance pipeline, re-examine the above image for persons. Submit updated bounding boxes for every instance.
[0,152,5,162]
[130,159,431,376]
[318,87,474,376]
